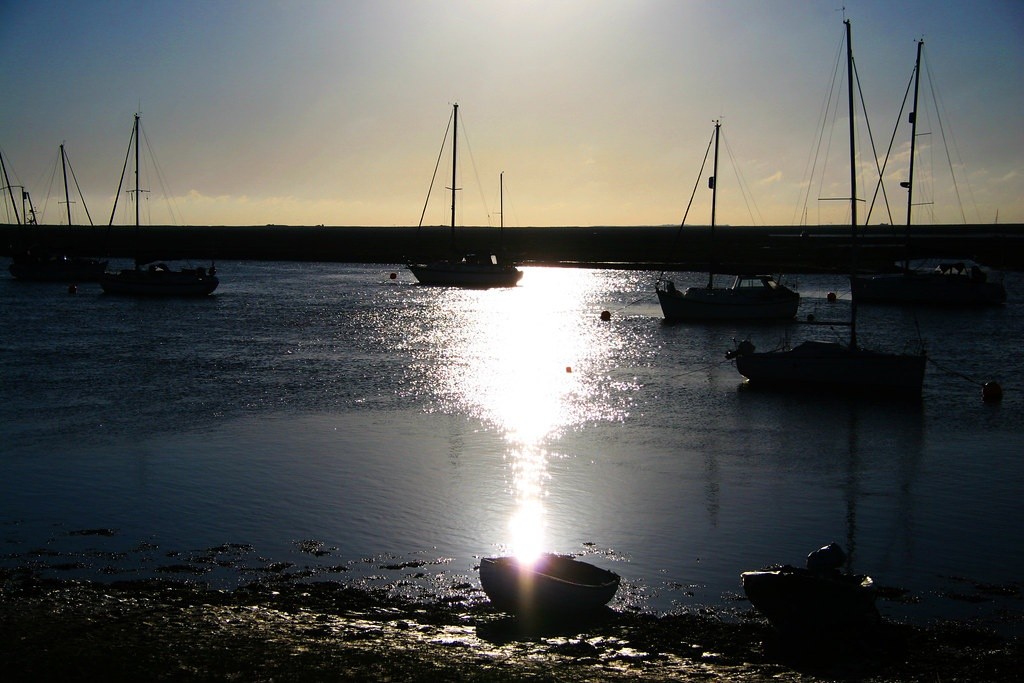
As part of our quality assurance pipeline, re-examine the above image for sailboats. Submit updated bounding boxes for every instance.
[402,102,527,292]
[85,112,221,300]
[0,143,111,287]
[654,131,803,330]
[731,21,927,404]
[849,34,1009,312]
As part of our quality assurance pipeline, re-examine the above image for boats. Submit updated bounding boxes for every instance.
[473,548,624,613]
[738,543,944,656]
